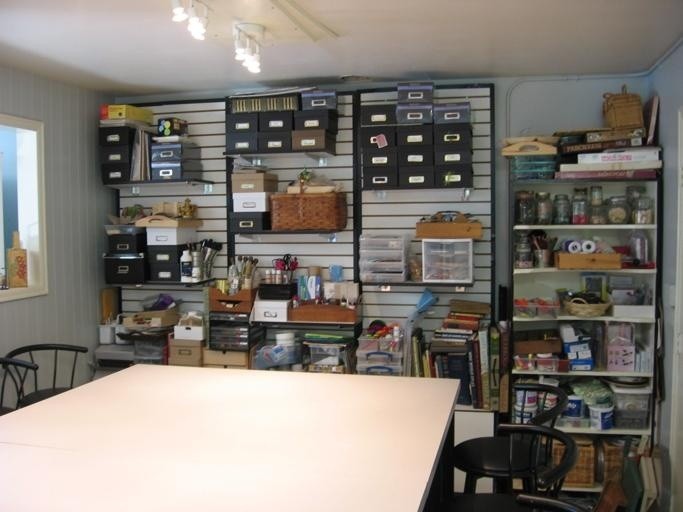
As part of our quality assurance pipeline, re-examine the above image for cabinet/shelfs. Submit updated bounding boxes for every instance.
[93,98,229,360]
[227,92,356,287]
[354,83,493,326]
[511,178,659,451]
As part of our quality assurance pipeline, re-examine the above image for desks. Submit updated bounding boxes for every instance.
[0,363,460,512]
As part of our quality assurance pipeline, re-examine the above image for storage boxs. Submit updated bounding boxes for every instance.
[209,315,265,348]
[231,173,278,193]
[397,126,434,145]
[99,105,155,125]
[100,127,139,145]
[103,255,150,283]
[360,251,407,260]
[258,112,293,131]
[360,261,407,272]
[434,104,471,124]
[397,105,434,126]
[202,348,251,369]
[226,114,258,131]
[361,166,399,188]
[357,365,404,377]
[229,212,272,231]
[361,236,407,250]
[357,329,404,352]
[436,145,473,165]
[293,130,337,153]
[360,273,407,281]
[151,144,202,160]
[259,284,298,301]
[291,303,354,323]
[101,164,132,183]
[360,105,397,125]
[106,235,146,254]
[147,246,195,262]
[305,343,353,367]
[398,83,434,105]
[362,146,398,166]
[150,263,181,281]
[423,238,472,282]
[151,162,202,180]
[226,133,258,153]
[399,166,435,188]
[233,192,271,212]
[271,192,348,230]
[356,352,404,365]
[208,288,256,313]
[145,221,198,244]
[435,166,473,188]
[99,147,134,163]
[253,339,302,372]
[398,145,435,165]
[435,125,472,145]
[173,325,209,340]
[302,92,338,109]
[231,96,301,112]
[158,119,188,135]
[360,128,397,145]
[603,94,644,128]
[293,110,340,130]
[259,133,292,153]
[254,301,288,322]
[135,340,167,358]
[169,340,204,366]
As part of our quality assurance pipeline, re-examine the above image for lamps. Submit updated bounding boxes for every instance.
[242,36,253,69]
[198,5,210,40]
[234,27,245,60]
[172,0,185,23]
[189,0,201,39]
[249,43,260,73]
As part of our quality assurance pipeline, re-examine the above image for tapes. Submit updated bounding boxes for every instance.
[562,240,580,254]
[215,279,230,295]
[581,240,598,253]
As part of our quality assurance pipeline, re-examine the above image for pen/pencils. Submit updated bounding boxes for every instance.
[529,234,559,250]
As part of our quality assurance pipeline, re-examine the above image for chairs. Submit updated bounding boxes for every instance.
[443,490,583,512]
[455,382,569,493]
[0,344,90,407]
[0,358,38,414]
[454,422,576,504]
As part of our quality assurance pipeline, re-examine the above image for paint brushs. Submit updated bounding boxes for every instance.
[192,240,199,251]
[231,251,259,279]
[188,240,193,252]
[201,239,205,253]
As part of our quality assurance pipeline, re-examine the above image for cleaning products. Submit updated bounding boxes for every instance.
[5,230,28,289]
